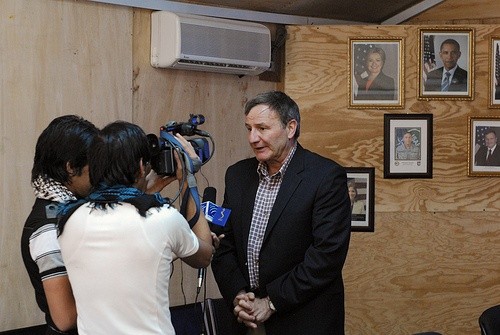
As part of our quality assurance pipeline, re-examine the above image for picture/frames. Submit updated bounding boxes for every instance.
[384,111,433,180]
[487,35,500,110]
[466,115,500,176]
[348,36,407,110]
[343,167,377,232]
[416,24,476,102]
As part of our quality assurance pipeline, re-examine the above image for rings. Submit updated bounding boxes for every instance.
[237,309,243,317]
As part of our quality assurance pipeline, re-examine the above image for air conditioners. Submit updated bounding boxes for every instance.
[150,10,273,77]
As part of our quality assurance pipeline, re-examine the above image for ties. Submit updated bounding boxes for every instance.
[488,149,491,159]
[442,71,451,90]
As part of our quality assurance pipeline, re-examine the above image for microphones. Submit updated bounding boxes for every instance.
[197,186,232,293]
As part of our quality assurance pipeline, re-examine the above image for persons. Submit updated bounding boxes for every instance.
[347,183,364,212]
[424,39,467,92]
[396,129,421,160]
[20,114,226,335]
[56,120,216,335]
[211,90,352,335]
[354,46,395,100]
[475,131,500,166]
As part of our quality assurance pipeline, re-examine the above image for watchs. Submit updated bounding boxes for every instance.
[267,297,277,312]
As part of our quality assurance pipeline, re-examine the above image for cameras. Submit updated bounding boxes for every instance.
[146,113,215,177]
